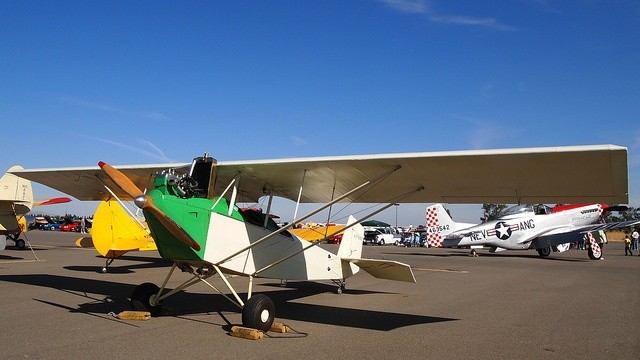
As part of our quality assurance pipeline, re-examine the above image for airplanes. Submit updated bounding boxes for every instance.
[428,197,637,260]
[77,195,354,276]
[6,139,630,342]
[0,164,72,254]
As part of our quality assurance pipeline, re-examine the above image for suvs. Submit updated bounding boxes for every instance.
[362,228,402,246]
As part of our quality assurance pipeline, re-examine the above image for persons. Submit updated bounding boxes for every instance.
[632,229,639,250]
[624,235,632,256]
[574,234,588,249]
[596,230,607,260]
